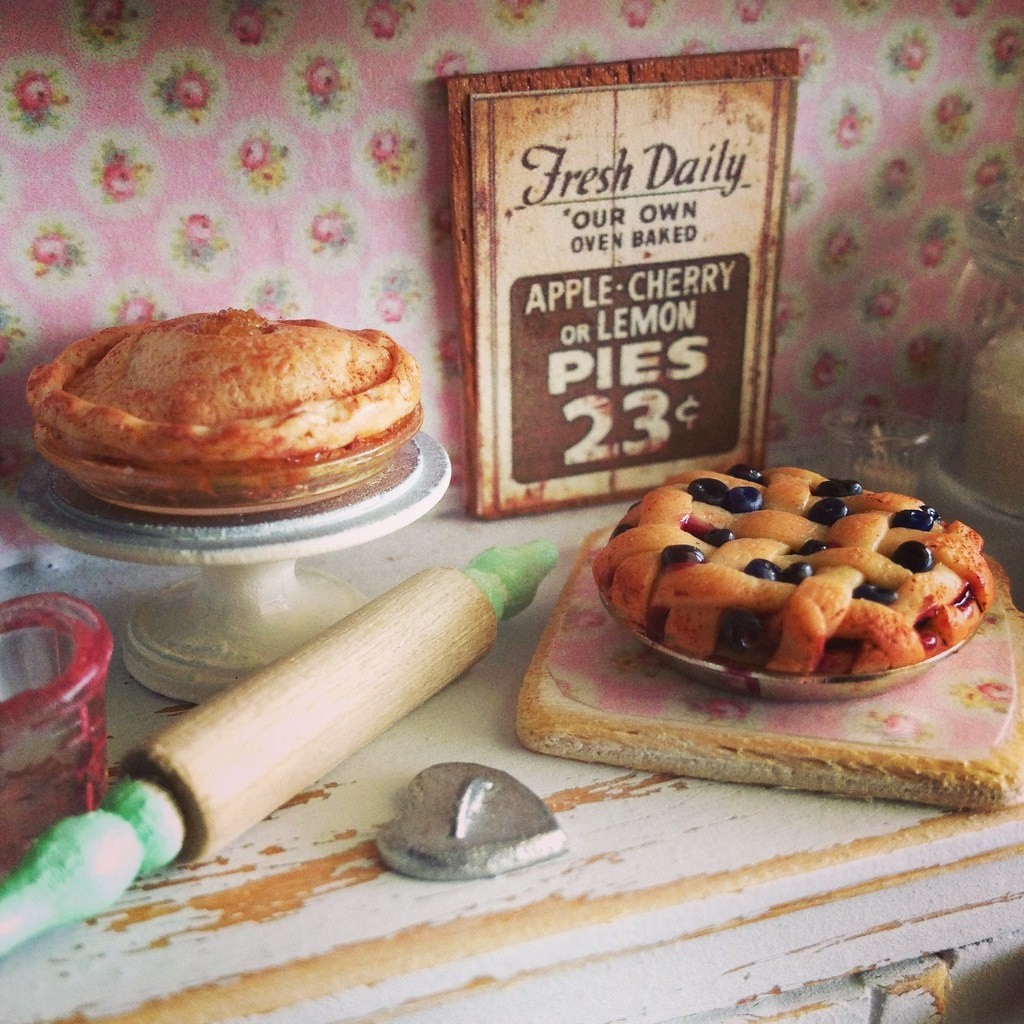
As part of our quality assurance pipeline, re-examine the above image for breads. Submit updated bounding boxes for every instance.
[591,463,995,675]
[24,306,423,507]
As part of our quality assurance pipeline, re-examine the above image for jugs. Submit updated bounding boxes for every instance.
[917,175,1024,532]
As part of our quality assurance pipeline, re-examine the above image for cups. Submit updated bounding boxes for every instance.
[812,407,935,498]
[0,591,114,884]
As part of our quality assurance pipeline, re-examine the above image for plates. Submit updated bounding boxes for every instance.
[596,587,985,701]
